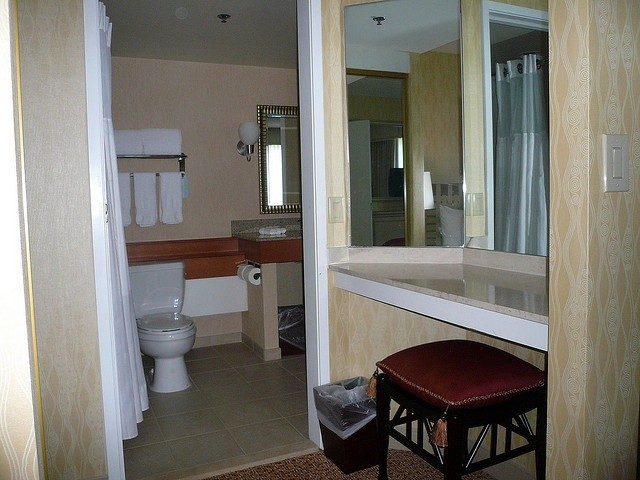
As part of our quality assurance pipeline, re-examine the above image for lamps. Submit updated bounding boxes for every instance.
[236,121,261,162]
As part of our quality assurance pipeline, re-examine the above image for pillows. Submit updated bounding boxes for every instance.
[439,203,465,247]
[366,339,547,448]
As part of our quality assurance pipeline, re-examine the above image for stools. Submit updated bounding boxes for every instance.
[374,372,546,480]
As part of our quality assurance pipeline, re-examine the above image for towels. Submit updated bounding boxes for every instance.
[133,172,158,228]
[118,170,132,228]
[114,128,182,156]
[158,171,184,224]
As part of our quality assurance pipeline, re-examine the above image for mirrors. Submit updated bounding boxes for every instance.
[257,105,302,215]
[343,1,465,248]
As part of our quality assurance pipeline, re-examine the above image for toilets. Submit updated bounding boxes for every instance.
[128,260,197,393]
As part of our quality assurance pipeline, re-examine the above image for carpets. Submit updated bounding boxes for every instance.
[202,448,498,480]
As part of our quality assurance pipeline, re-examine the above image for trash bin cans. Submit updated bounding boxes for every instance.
[312,376,379,475]
